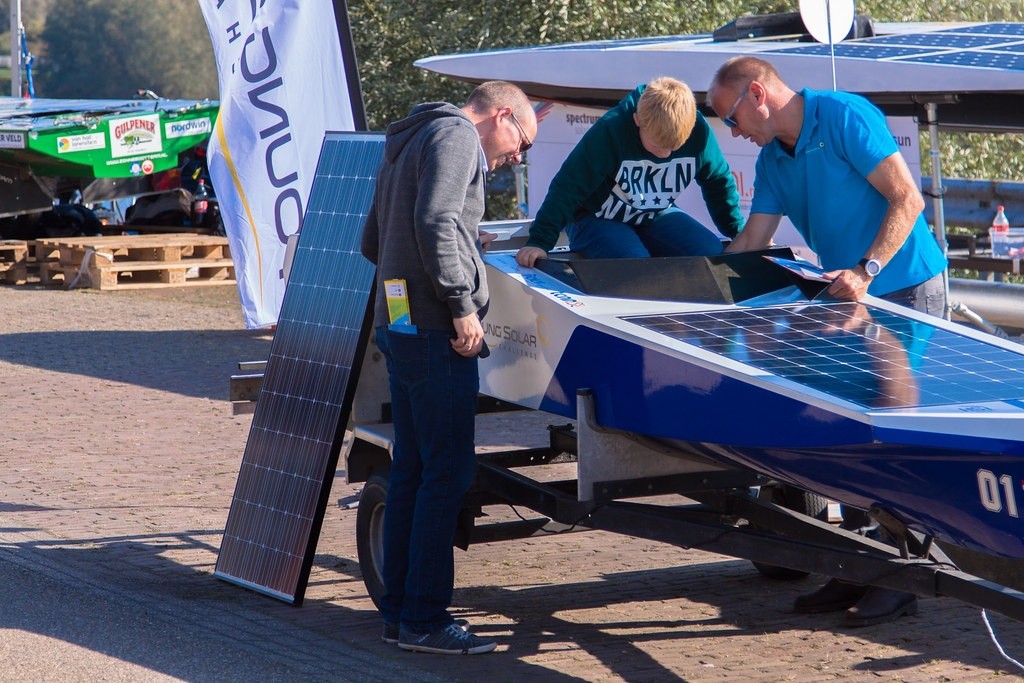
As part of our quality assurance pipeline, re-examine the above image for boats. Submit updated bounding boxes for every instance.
[407,219,1023,564]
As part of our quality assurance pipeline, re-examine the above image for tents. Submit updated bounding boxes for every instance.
[412,21,1024,344]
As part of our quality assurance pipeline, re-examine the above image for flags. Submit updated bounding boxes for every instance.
[204,0,368,330]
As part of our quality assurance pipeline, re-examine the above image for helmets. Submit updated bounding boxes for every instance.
[56,176,81,194]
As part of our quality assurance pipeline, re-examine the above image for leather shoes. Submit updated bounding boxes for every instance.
[795,575,872,613]
[841,585,918,626]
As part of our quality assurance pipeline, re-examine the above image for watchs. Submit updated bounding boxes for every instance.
[858,258,881,277]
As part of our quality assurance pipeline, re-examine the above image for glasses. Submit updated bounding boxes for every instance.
[722,81,752,127]
[499,107,533,152]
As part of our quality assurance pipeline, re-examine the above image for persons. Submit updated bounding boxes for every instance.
[360,80,537,654]
[515,74,775,270]
[706,54,950,326]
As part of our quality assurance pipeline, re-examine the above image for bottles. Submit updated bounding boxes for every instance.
[992,205,1009,258]
[194,179,209,226]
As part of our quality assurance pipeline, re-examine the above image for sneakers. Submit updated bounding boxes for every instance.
[398,618,496,655]
[380,617,470,644]
